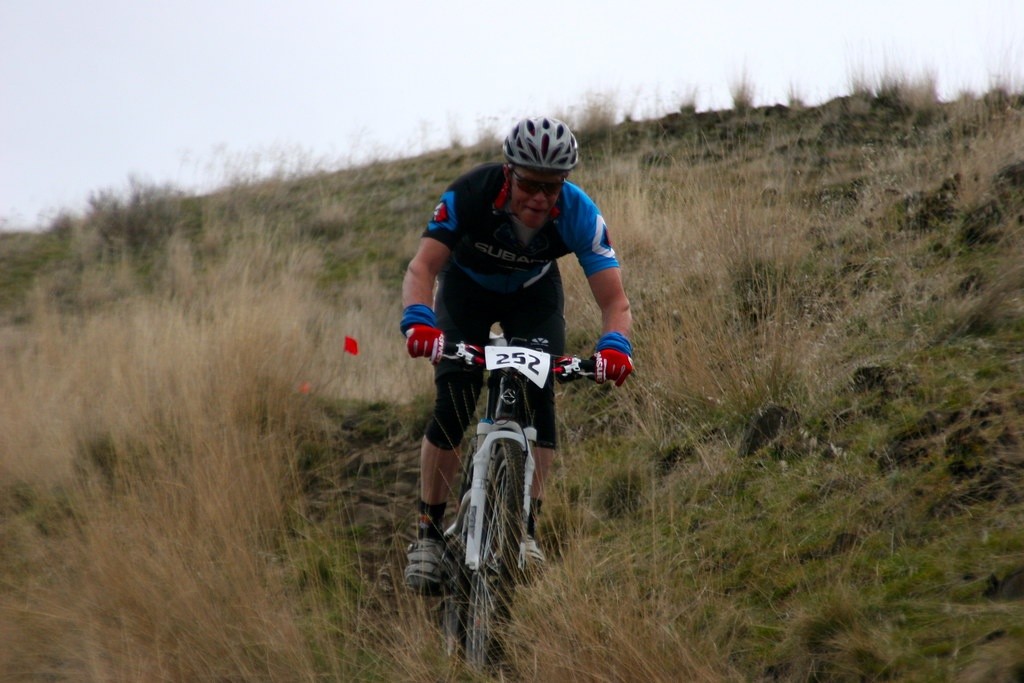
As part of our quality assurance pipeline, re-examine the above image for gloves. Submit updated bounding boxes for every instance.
[586,348,634,389]
[404,323,445,367]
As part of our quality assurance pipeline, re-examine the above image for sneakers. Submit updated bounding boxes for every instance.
[519,533,546,581]
[402,535,445,596]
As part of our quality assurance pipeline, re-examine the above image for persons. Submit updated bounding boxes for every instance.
[399,121,636,599]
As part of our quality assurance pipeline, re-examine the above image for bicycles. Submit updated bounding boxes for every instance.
[407,333,635,677]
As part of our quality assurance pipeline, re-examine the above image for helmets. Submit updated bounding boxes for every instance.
[501,115,581,174]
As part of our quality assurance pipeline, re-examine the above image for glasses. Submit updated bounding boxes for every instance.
[510,170,565,198]
